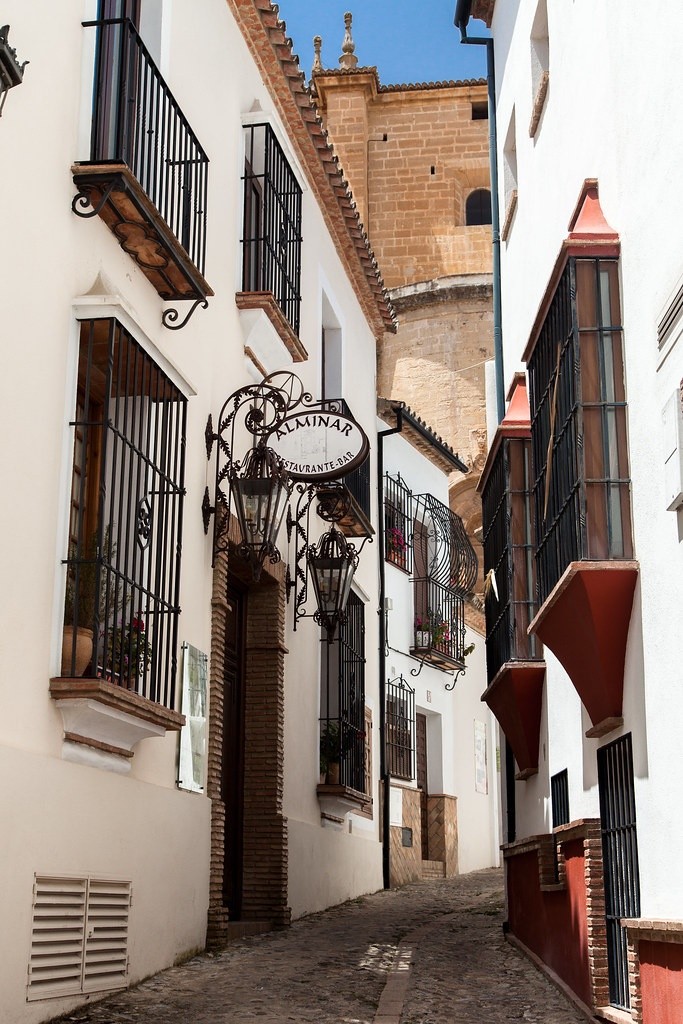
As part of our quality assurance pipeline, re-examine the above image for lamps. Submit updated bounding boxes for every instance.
[285,481,373,646]
[201,384,291,584]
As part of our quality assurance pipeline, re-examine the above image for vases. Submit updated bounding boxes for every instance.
[415,630,433,646]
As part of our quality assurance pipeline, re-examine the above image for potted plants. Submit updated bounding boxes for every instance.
[320,718,354,785]
[62,519,129,677]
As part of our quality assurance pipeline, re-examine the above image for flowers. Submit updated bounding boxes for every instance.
[386,527,406,555]
[414,607,441,629]
[434,622,449,645]
[99,610,152,679]
